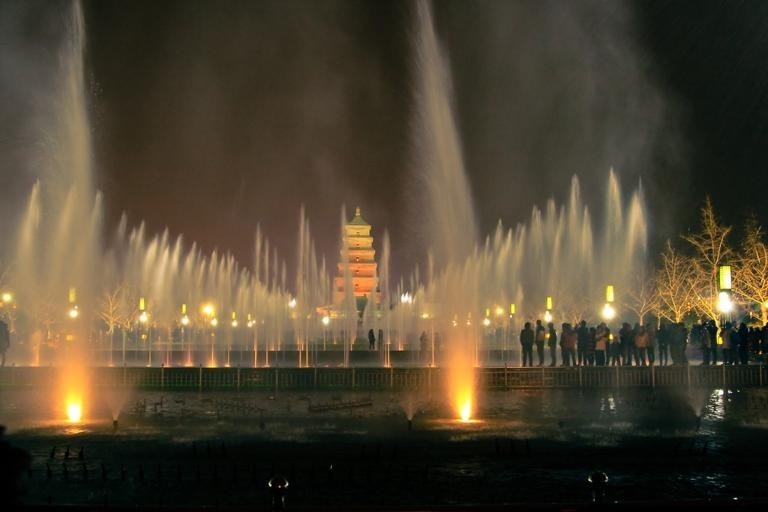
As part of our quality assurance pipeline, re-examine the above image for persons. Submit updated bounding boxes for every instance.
[520,318,767,369]
[378,328,383,350]
[367,326,376,350]
[434,332,442,353]
[419,331,429,353]
